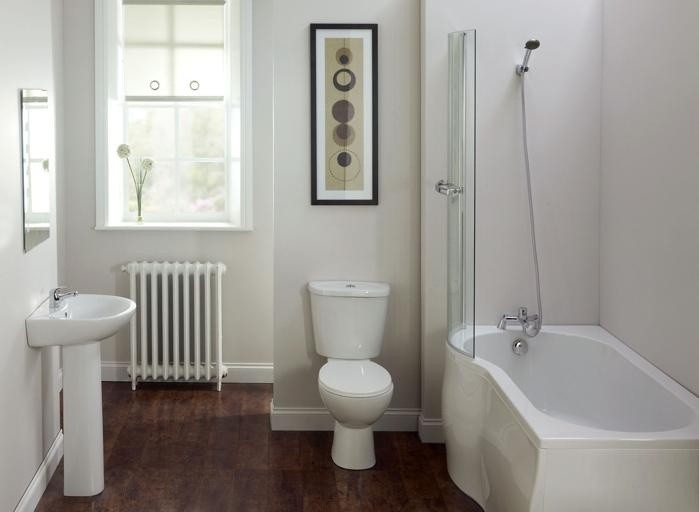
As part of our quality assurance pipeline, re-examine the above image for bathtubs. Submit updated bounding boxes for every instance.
[442,322,699,512]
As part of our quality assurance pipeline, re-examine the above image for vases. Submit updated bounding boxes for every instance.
[136,187,143,222]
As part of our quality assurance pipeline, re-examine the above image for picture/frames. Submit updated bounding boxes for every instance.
[310,21,378,205]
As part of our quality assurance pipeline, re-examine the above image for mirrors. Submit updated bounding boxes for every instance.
[20,86,53,252]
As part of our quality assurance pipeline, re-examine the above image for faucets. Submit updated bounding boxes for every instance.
[518,306,538,339]
[49,287,78,309]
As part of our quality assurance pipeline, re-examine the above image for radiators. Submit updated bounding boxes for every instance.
[119,257,230,392]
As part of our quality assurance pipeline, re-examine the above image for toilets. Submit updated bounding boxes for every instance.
[306,279,393,470]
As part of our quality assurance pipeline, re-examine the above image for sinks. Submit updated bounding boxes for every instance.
[27,292,137,347]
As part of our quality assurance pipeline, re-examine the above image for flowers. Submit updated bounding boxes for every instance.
[117,143,153,217]
